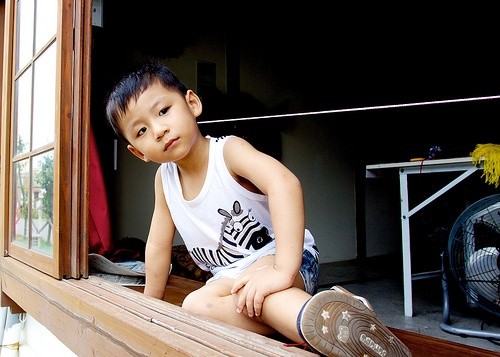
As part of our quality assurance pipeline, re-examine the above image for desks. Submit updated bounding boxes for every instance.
[365,155,493,317]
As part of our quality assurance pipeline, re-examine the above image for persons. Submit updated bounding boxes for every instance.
[105,62,413,357]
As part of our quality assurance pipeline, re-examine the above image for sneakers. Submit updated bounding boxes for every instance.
[297,288,412,356]
